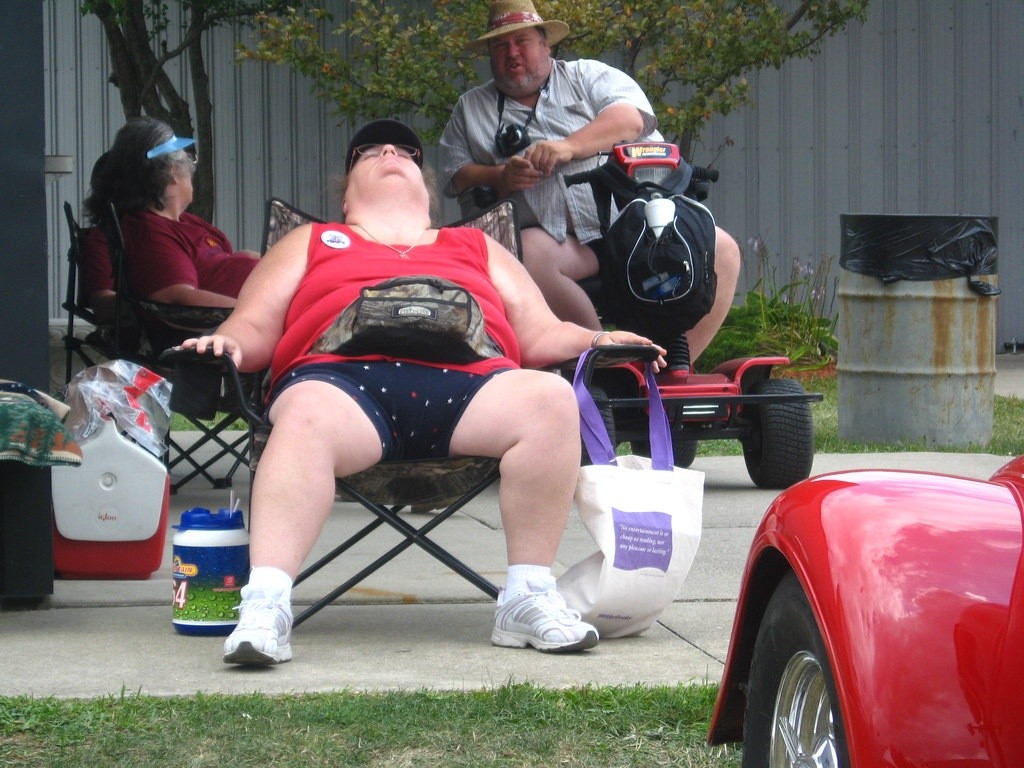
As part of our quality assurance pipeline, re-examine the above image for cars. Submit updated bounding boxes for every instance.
[705,453,1024,768]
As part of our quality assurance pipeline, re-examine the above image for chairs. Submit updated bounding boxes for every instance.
[160,179,664,621]
[54,188,259,493]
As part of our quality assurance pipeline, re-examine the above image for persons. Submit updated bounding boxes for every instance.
[81,114,473,514]
[436,0,742,369]
[181,117,668,666]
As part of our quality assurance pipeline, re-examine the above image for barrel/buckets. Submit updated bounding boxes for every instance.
[835,213,999,444]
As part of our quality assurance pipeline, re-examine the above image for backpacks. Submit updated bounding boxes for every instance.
[592,156,716,339]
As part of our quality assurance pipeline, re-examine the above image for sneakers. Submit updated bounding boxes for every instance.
[491,575,599,651]
[224,585,293,664]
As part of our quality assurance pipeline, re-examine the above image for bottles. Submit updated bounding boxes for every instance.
[171,507,250,635]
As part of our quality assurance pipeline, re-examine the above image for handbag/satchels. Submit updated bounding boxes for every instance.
[312,276,484,357]
[556,345,704,640]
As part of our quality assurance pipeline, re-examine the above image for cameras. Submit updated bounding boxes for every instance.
[496,123,530,156]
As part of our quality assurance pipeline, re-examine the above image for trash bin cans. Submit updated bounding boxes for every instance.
[835,213,996,452]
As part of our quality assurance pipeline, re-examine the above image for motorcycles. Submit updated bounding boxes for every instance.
[553,141,827,491]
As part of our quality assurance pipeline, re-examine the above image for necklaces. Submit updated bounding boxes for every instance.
[357,224,425,258]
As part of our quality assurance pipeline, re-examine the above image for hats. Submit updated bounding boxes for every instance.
[346,120,423,170]
[146,134,196,158]
[466,0,569,55]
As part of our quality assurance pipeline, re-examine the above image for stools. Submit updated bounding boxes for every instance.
[0,378,71,610]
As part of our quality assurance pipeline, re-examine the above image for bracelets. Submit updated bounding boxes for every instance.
[591,330,610,348]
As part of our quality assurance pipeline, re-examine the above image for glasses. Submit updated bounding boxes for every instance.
[187,152,198,164]
[350,143,420,172]
